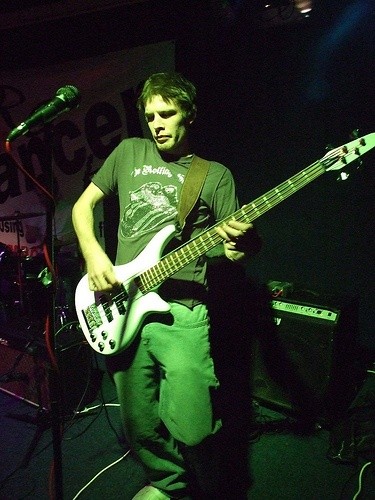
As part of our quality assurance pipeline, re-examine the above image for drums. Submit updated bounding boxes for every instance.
[53,308,90,355]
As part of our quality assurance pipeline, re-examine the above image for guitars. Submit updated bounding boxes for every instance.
[73,129,374,357]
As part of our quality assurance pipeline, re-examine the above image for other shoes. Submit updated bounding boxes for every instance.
[131,486,174,500]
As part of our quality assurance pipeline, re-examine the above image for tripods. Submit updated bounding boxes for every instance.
[3,154,99,474]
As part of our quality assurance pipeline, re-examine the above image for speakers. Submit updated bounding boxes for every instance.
[247,277,361,423]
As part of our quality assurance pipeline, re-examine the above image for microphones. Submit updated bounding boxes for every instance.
[6,85,81,142]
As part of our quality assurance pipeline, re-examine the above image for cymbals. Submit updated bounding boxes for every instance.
[0,212,45,220]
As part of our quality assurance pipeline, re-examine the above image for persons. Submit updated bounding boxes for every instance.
[24,172,86,277]
[69,69,255,500]
